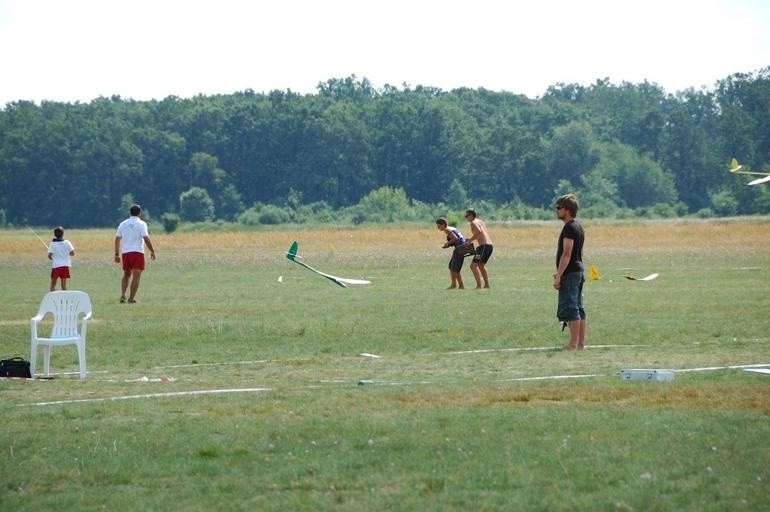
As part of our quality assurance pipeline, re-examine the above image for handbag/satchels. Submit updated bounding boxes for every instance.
[0,356,31,378]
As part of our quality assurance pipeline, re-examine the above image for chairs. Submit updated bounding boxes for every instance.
[29,290,92,379]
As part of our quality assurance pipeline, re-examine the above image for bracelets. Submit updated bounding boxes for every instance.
[114,252,119,257]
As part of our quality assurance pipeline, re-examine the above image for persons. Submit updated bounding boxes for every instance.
[113,204,155,304]
[435,219,467,289]
[549,194,588,351]
[47,225,74,292]
[463,210,493,288]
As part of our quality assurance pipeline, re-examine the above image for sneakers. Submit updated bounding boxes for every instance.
[120,297,126,303]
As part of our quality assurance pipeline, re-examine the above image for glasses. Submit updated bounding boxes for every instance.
[557,207,564,210]
